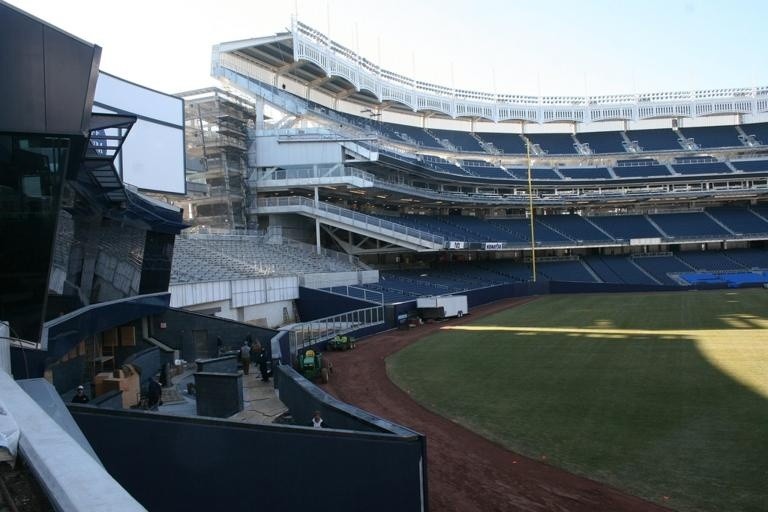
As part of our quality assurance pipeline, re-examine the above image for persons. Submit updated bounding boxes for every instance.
[147,376,162,412]
[307,408,330,428]
[238,333,269,381]
[71,385,89,404]
[215,335,224,358]
